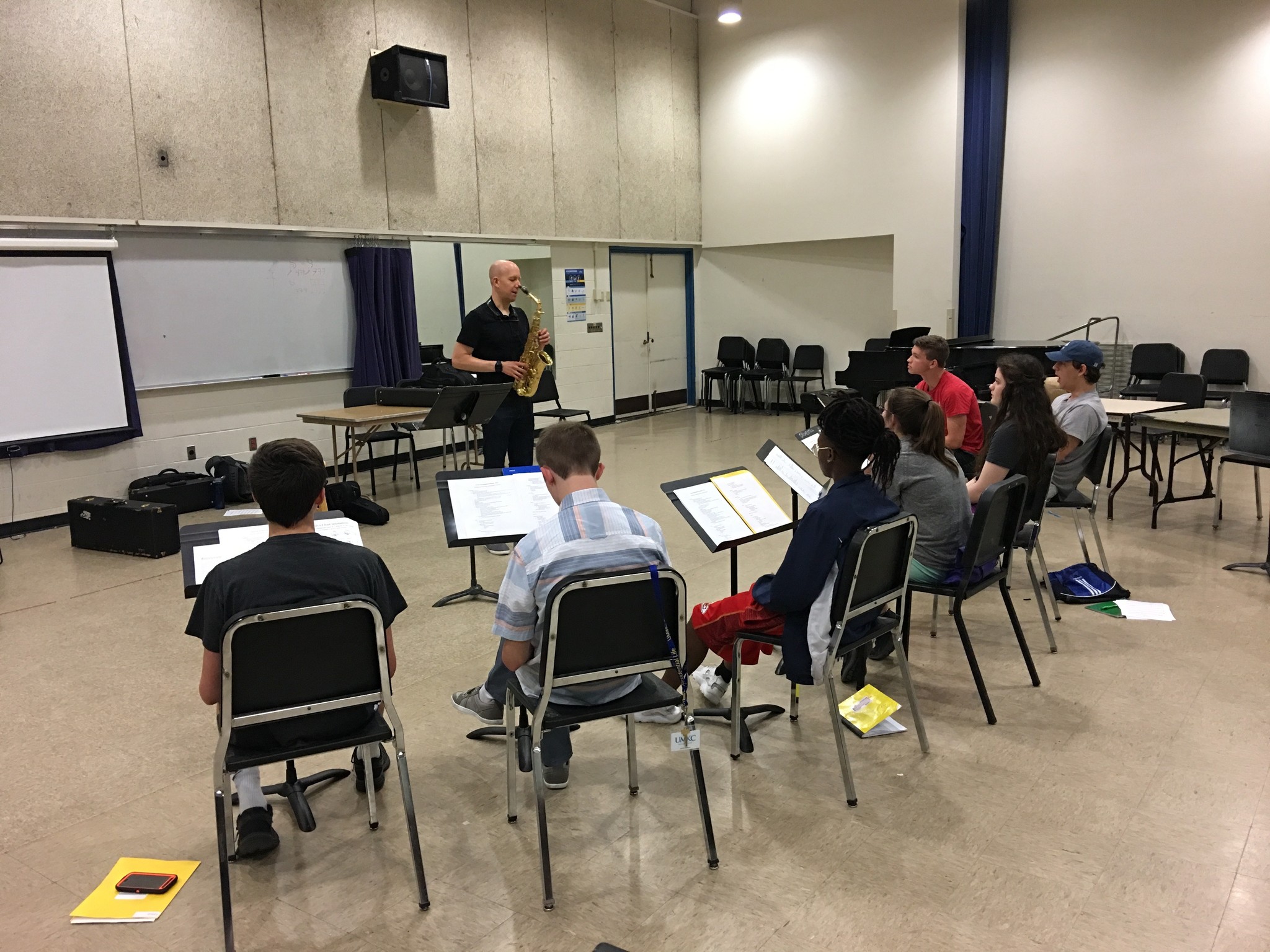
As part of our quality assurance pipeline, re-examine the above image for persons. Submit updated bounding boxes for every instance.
[184,438,408,857]
[452,260,550,555]
[623,400,902,724]
[966,352,1068,556]
[451,420,672,790]
[1044,340,1110,497]
[840,387,974,683]
[908,336,984,480]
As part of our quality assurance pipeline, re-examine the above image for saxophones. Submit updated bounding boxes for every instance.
[512,284,557,399]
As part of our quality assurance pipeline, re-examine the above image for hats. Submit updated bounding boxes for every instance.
[1045,340,1104,370]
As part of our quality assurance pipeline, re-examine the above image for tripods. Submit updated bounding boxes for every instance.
[659,464,788,752]
[424,380,513,611]
[173,509,351,833]
[433,470,580,772]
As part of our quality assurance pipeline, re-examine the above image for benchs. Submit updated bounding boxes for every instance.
[800,388,859,430]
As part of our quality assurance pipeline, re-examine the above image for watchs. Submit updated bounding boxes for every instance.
[495,361,503,373]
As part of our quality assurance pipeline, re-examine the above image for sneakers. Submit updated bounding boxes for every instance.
[621,704,682,724]
[691,664,731,706]
[484,543,510,555]
[234,803,280,857]
[451,683,504,725]
[541,758,570,789]
[351,740,391,793]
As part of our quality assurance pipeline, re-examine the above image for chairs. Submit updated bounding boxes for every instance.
[396,378,459,479]
[729,512,932,808]
[964,402,998,479]
[928,450,1063,654]
[701,336,824,415]
[214,594,431,952]
[533,370,591,446]
[1005,423,1112,590]
[1107,342,1270,529]
[506,566,725,909]
[342,385,421,496]
[856,474,1040,724]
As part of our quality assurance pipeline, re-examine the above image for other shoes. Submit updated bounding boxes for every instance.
[868,607,903,661]
[840,639,874,685]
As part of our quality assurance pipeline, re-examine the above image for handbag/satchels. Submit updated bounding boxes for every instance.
[1039,562,1131,605]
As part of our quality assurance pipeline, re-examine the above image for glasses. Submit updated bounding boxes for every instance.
[811,443,833,459]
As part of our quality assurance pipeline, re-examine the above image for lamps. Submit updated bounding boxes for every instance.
[714,0,742,23]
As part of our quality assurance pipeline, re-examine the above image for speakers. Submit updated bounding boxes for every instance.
[367,44,450,110]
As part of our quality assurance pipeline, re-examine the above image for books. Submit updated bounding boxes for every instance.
[829,683,908,739]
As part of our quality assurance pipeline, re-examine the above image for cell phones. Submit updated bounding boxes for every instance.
[115,871,179,895]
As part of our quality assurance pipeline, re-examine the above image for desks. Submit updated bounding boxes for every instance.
[296,403,486,501]
[1099,398,1187,520]
[1133,407,1231,528]
